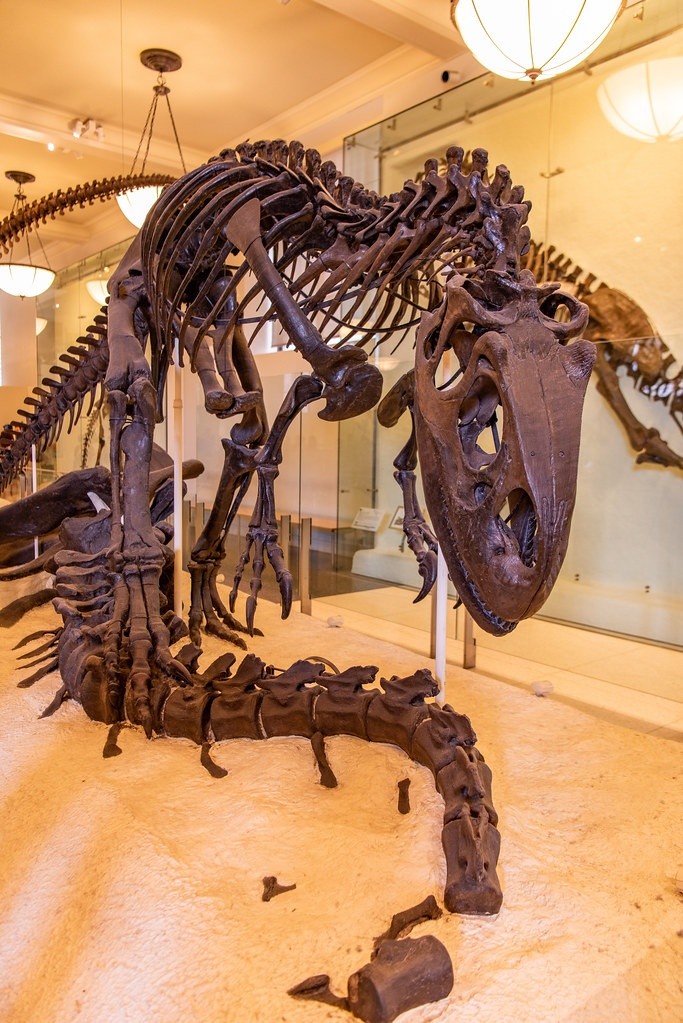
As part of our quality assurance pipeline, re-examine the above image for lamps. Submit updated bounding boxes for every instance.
[0,170,57,301]
[86,279,109,306]
[597,57,683,142]
[451,0,626,86]
[36,297,48,336]
[115,48,187,227]
